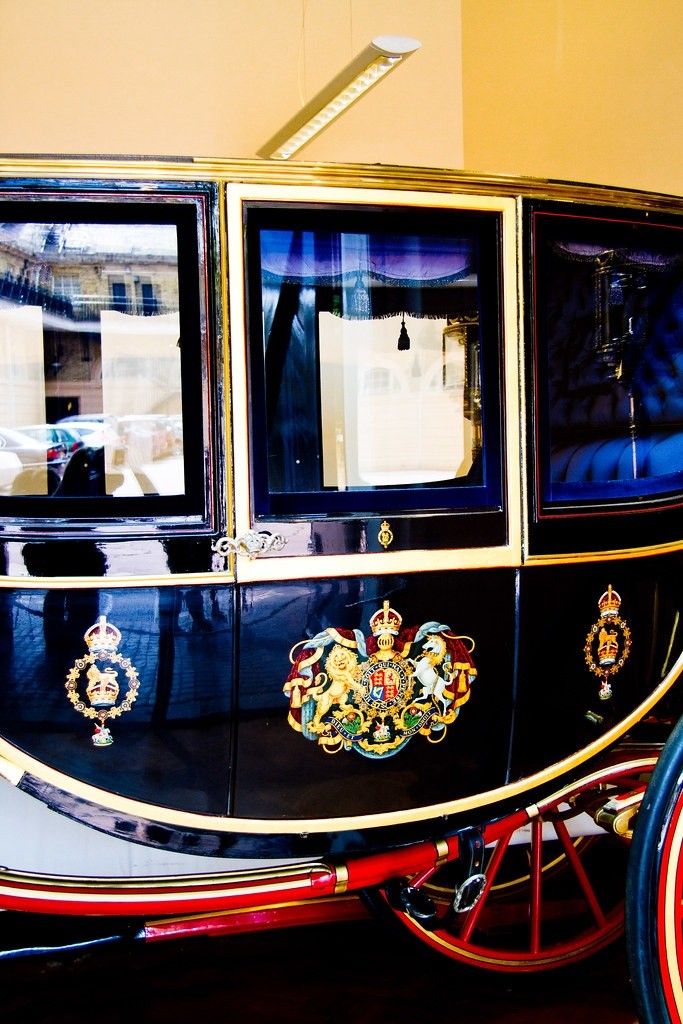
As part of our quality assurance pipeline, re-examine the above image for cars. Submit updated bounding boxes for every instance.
[3,409,180,492]
[1,146,683,973]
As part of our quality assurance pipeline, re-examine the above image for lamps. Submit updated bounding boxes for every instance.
[255,1,423,162]
